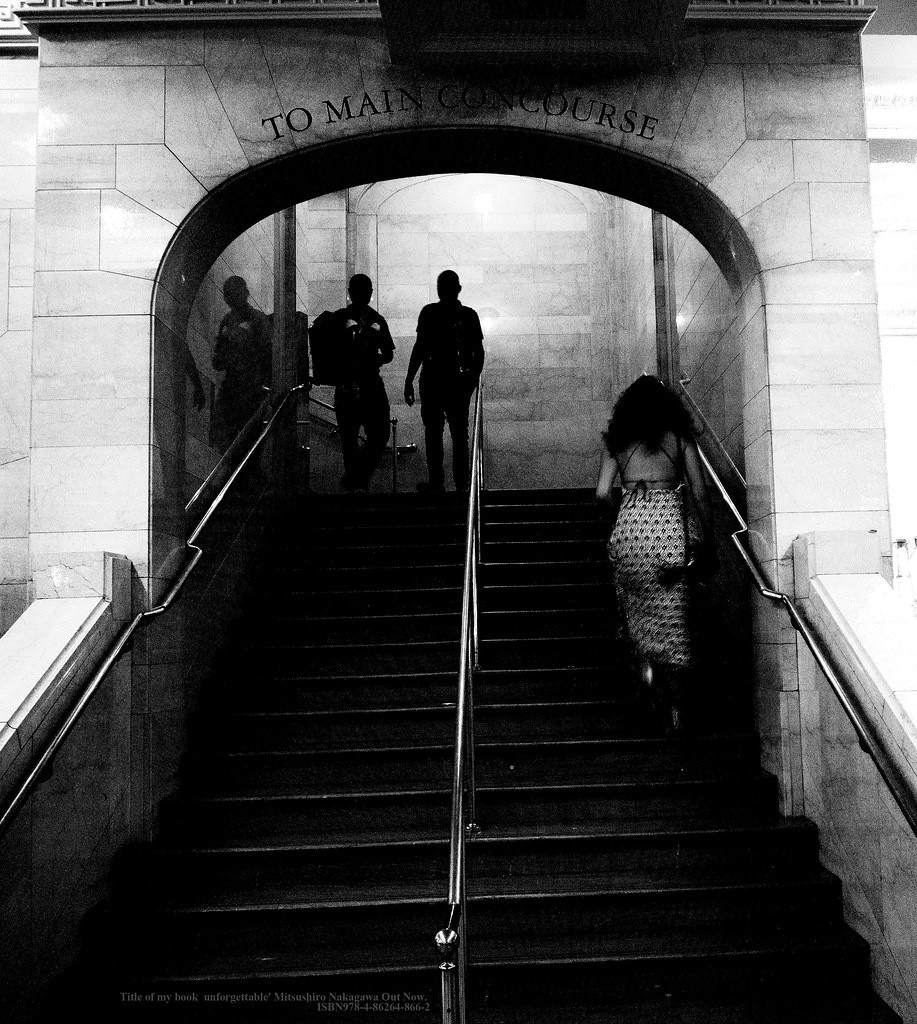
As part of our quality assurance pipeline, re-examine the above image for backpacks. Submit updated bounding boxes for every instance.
[310,311,370,385]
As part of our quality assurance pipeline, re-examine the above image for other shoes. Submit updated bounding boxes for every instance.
[455,484,469,498]
[416,481,446,492]
[663,706,686,740]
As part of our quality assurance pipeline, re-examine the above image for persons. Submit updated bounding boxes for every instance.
[595,372,712,731]
[403,270,485,493]
[320,274,396,496]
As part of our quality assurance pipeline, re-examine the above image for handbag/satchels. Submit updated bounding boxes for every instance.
[675,433,704,546]
